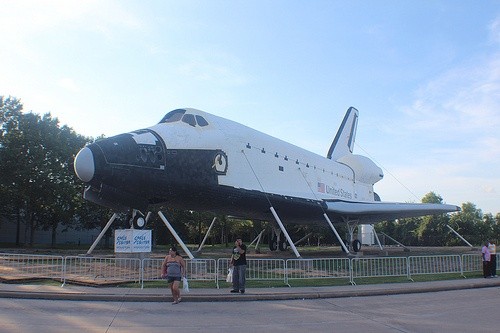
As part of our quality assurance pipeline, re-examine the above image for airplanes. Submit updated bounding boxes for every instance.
[73,105,461,253]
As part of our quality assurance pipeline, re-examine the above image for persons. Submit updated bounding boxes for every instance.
[162,247,185,304]
[231,238,247,293]
[482,239,497,278]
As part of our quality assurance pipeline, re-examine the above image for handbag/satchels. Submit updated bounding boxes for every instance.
[163,264,167,274]
[183,277,189,292]
[226,268,232,283]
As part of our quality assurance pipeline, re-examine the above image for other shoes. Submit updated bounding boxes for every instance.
[241,290,244,293]
[172,301,178,304]
[231,289,239,293]
[178,297,183,302]
